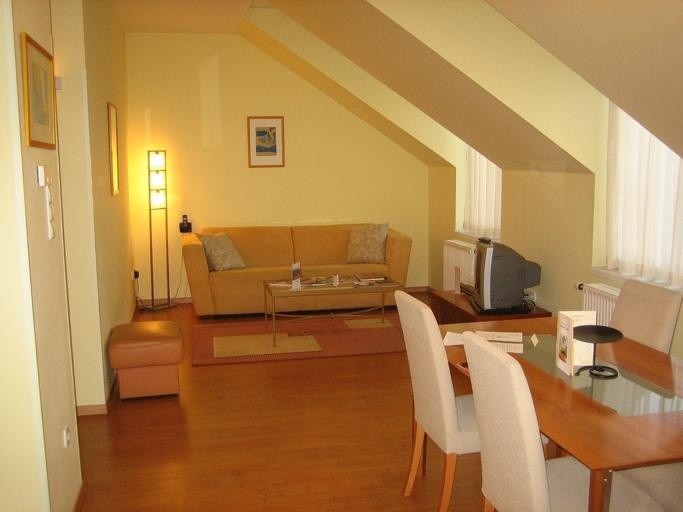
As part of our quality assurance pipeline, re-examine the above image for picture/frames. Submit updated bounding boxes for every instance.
[246,115,285,168]
[18,32,55,150]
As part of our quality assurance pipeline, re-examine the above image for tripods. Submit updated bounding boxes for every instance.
[469,241,541,314]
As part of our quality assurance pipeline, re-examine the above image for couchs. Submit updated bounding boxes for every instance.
[180,223,412,318]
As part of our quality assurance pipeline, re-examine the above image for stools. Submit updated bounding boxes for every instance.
[105,321,186,400]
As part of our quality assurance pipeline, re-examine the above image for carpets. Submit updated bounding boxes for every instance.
[190,314,406,367]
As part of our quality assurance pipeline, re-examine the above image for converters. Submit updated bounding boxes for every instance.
[135,271,139,278]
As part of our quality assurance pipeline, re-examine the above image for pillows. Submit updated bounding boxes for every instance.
[346,224,388,266]
[194,231,246,272]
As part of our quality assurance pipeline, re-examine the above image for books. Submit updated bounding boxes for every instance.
[354,271,386,281]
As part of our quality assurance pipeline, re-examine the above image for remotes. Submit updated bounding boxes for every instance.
[478,237,491,243]
